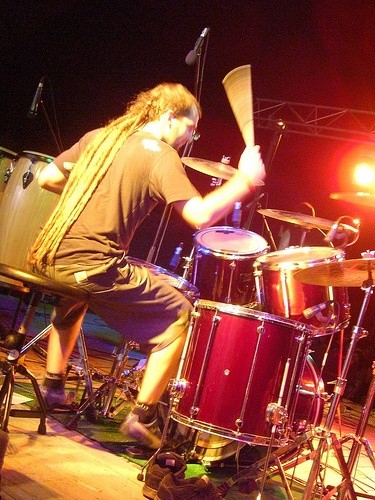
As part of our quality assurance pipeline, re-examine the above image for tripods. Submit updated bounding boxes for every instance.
[19,36,208,429]
[216,279,375,500]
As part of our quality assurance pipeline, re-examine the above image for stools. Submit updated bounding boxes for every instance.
[0,264,98,435]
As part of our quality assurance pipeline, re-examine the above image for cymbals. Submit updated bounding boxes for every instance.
[181,157,265,186]
[257,209,359,233]
[293,258,375,287]
[329,191,375,207]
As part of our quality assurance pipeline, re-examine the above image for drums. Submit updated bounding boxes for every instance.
[125,257,200,349]
[0,151,78,292]
[171,299,315,447]
[0,147,18,196]
[157,353,325,465]
[192,226,270,309]
[253,246,351,336]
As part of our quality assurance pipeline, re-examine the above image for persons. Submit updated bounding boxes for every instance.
[24,82,267,449]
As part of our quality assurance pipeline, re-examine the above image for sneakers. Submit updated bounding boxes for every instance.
[142,450,225,500]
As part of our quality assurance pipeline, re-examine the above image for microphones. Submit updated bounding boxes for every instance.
[185,28,208,66]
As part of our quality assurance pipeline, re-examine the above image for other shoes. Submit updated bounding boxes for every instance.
[38,383,78,407]
[121,402,163,449]
